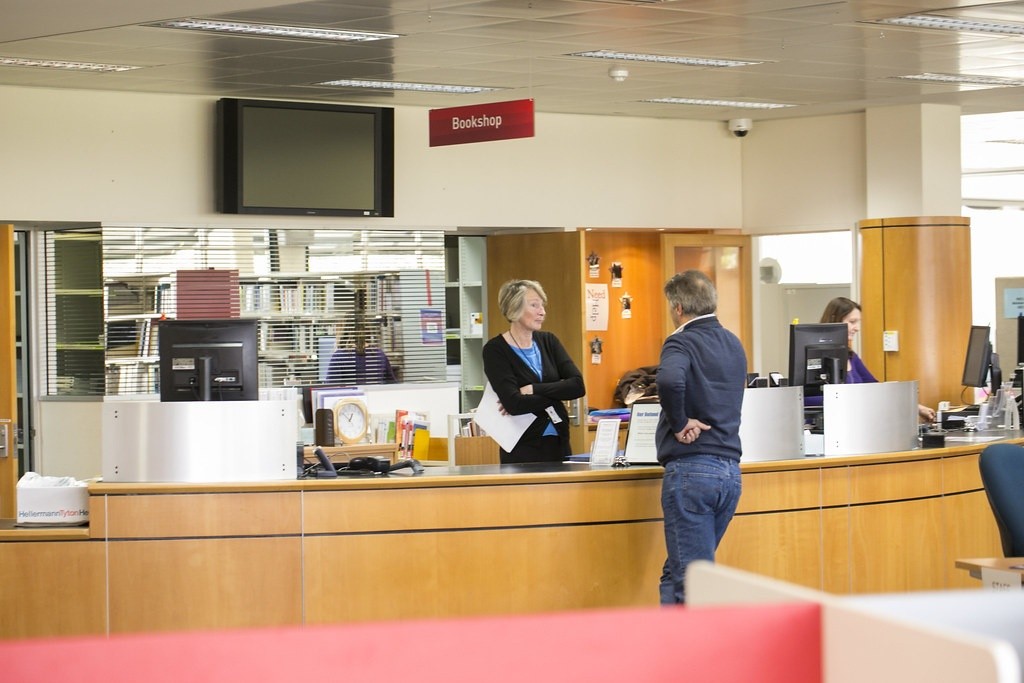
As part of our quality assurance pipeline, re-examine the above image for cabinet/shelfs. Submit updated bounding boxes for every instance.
[390,269,446,382]
[108,272,405,386]
[56,233,106,351]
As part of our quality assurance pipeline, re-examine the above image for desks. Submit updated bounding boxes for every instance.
[955,557,1024,586]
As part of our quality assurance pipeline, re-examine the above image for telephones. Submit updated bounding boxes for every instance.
[348,455,391,474]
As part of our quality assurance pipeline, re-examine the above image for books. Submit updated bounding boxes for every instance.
[370,409,429,461]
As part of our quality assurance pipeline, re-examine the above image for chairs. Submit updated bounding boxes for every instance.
[979,443,1024,558]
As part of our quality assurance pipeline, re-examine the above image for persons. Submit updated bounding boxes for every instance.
[804,297,935,424]
[325,310,397,384]
[655,270,747,604]
[483,280,586,465]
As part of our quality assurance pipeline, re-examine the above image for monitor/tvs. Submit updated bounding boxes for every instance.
[219,98,394,218]
[790,323,848,396]
[158,319,258,402]
[961,326,990,386]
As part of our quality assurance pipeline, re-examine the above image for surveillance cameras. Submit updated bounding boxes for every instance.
[728,119,752,137]
